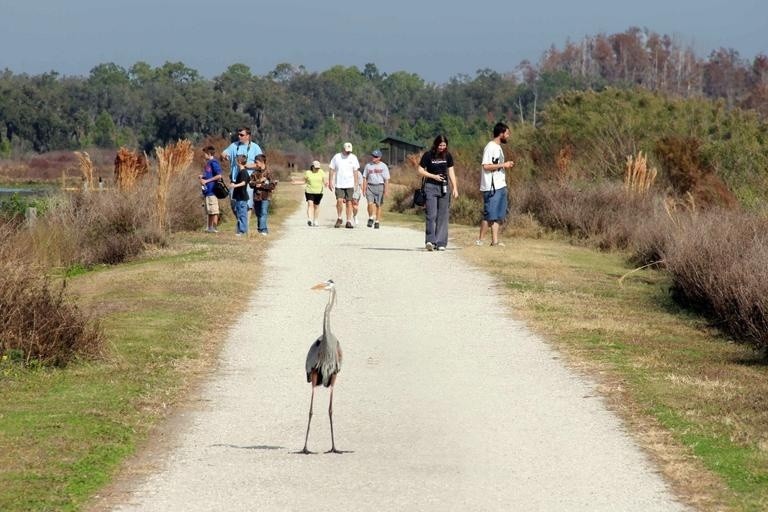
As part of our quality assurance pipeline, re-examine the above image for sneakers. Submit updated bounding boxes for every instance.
[489,243,505,247]
[366,218,374,227]
[203,227,218,233]
[346,222,354,229]
[475,240,484,246]
[308,220,319,227]
[437,246,445,251]
[426,242,436,252]
[235,230,267,237]
[374,222,380,229]
[334,219,343,229]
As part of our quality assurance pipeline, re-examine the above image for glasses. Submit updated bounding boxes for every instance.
[238,133,248,137]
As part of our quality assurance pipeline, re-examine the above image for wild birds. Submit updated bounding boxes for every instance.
[289,279,353,454]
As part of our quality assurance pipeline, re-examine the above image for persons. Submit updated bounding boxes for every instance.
[416,134,459,250]
[226,153,248,235]
[342,161,364,225]
[248,155,278,234]
[219,127,261,229]
[474,121,515,246]
[291,160,330,227]
[197,144,222,234]
[361,147,390,228]
[328,142,359,228]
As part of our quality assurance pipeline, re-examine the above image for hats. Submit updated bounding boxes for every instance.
[343,142,353,153]
[371,150,382,157]
[312,160,321,169]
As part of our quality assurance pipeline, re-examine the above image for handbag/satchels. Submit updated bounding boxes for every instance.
[413,188,427,207]
[211,181,230,200]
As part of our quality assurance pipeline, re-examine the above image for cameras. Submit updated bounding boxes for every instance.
[439,172,448,194]
